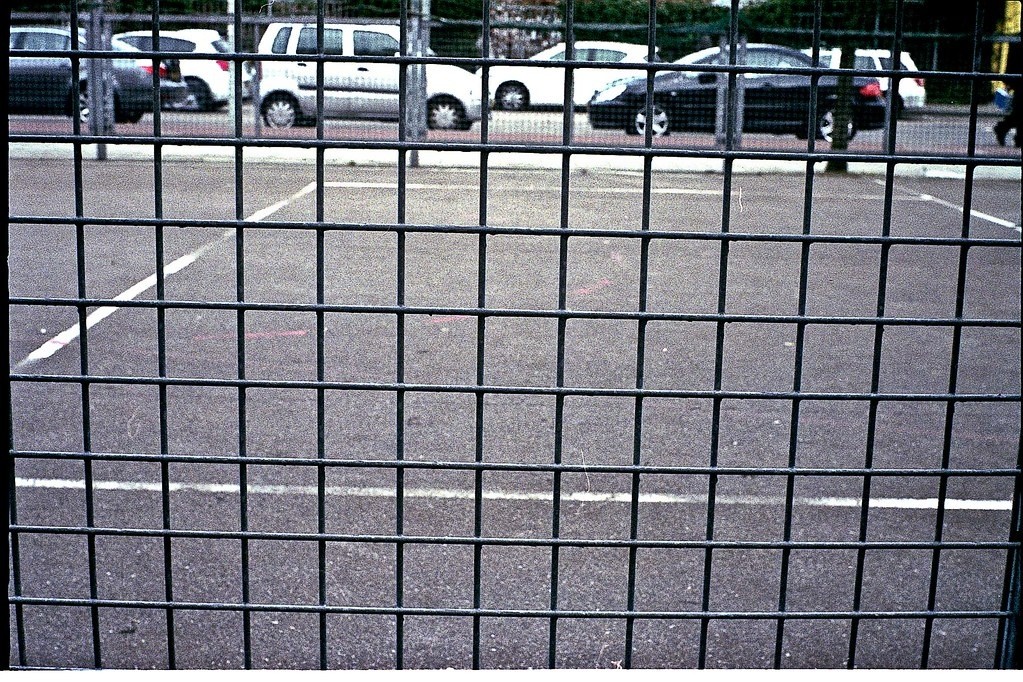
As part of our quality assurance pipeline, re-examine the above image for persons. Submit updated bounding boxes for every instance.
[994,33,1022,147]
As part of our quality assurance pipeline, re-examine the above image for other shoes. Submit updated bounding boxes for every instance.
[994,122,1007,146]
[1014,134,1023,147]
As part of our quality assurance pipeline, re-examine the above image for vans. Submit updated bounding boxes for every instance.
[254,21,481,131]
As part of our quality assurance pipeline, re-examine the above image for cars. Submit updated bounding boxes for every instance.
[781,48,927,109]
[10,26,167,125]
[113,28,232,110]
[587,42,887,142]
[474,41,666,111]
